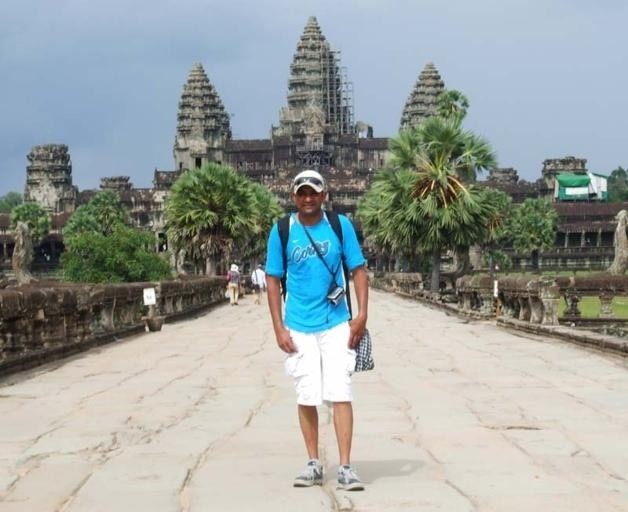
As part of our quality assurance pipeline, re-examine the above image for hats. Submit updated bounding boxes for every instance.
[231,264,238,271]
[294,170,329,192]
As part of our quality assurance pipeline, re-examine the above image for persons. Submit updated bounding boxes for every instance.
[266,169,369,491]
[227,263,241,306]
[250,264,266,303]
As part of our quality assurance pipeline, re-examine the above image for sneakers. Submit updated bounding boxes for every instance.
[336,467,364,490]
[294,461,323,487]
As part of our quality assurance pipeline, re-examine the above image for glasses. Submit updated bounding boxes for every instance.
[294,177,323,186]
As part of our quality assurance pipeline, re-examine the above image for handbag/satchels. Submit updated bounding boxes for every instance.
[356,328,374,371]
[225,291,230,298]
[251,284,259,289]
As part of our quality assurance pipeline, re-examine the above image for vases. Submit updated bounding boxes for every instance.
[146,316,163,331]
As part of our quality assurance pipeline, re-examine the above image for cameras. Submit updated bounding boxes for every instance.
[325,284,347,307]
